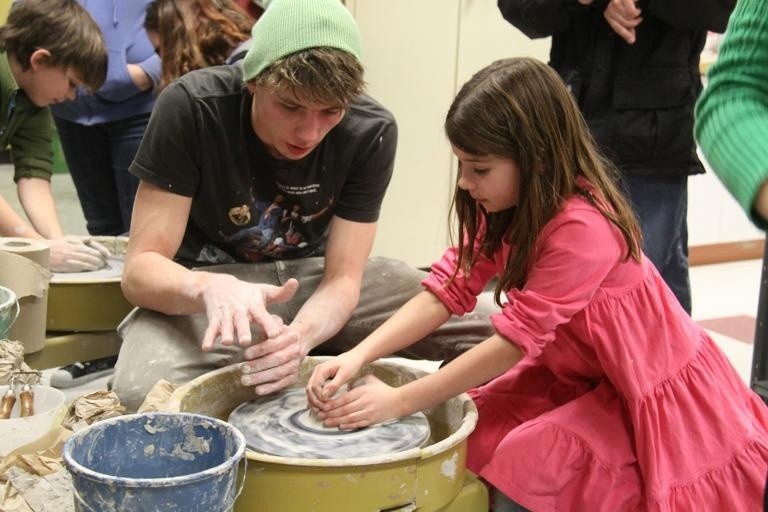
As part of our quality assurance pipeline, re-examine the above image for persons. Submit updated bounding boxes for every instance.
[140,0,253,88]
[1,0,110,275]
[493,2,734,319]
[306,54,767,512]
[108,0,508,425]
[42,0,165,390]
[692,2,766,401]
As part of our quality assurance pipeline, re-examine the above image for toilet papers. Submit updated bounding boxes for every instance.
[0,236,51,355]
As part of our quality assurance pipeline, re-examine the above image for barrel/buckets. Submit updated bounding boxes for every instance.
[62,410,248,511]
[0,285,20,340]
[0,384,65,458]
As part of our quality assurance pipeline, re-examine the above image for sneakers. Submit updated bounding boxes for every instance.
[50,356,117,389]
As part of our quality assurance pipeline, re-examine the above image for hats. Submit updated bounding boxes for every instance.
[241,0,363,83]
